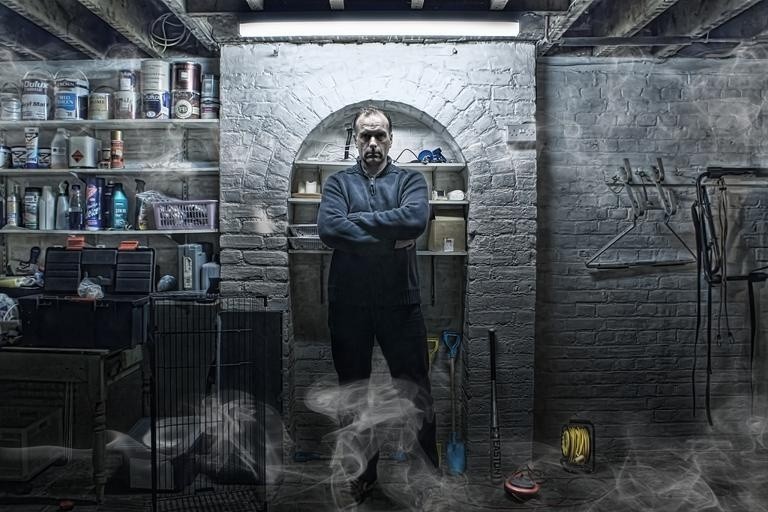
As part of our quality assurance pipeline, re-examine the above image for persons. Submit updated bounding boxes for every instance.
[315,104,441,507]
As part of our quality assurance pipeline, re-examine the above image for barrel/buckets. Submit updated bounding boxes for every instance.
[20,66,55,120]
[53,66,90,119]
[0,82,22,121]
[88,84,115,119]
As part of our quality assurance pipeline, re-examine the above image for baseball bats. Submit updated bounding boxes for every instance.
[489,329,503,485]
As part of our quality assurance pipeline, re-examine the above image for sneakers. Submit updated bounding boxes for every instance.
[414,486,449,512]
[333,469,378,509]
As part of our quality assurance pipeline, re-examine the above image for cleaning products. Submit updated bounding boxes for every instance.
[135,178,153,230]
[56,181,70,230]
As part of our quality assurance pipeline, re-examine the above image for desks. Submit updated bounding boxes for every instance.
[1,335,151,506]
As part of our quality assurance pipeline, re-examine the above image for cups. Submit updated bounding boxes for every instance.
[0,145,26,169]
[38,147,51,169]
[297,180,320,193]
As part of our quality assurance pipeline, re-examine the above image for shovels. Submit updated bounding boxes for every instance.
[426,330,466,474]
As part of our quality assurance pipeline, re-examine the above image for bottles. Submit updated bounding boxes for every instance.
[0,179,55,231]
[70,177,128,229]
[50,127,67,168]
[109,128,124,169]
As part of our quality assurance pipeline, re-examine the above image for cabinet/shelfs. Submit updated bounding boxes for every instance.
[0,116,219,234]
[285,159,466,256]
